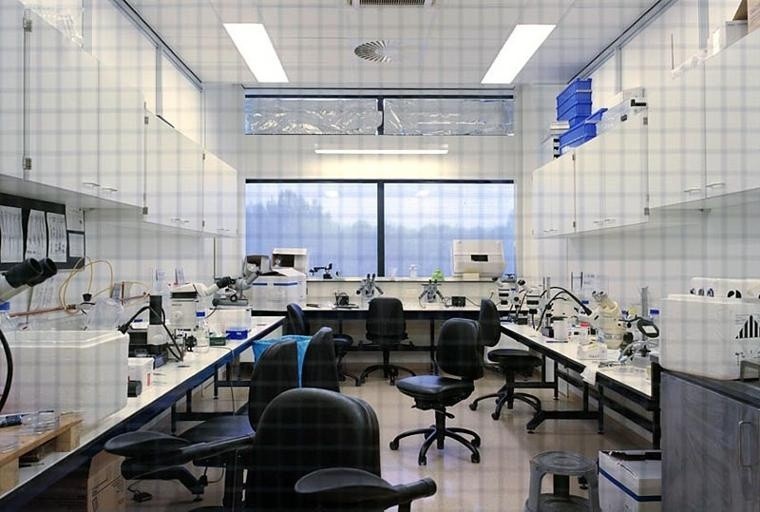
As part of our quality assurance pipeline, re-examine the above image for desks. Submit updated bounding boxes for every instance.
[546,338,659,451]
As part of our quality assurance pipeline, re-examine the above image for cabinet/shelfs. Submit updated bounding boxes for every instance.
[83,111,202,239]
[659,367,759,512]
[576,109,698,236]
[649,26,760,210]
[201,146,239,239]
[530,148,582,244]
[1,0,23,198]
[23,8,147,211]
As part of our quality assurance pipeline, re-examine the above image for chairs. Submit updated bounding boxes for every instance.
[176,297,437,512]
[390,318,484,465]
[479,298,545,420]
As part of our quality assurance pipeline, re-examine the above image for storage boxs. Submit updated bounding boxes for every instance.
[0,328,131,428]
[28,448,129,512]
[597,449,663,512]
[127,355,156,390]
[706,19,747,58]
[536,74,648,169]
[657,293,759,383]
[245,246,309,311]
[731,0,760,35]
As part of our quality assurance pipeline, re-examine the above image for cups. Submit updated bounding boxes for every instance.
[552,320,568,340]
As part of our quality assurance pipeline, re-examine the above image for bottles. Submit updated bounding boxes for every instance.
[579,300,591,314]
[648,309,658,323]
[409,265,418,279]
[0,301,18,332]
[193,311,210,353]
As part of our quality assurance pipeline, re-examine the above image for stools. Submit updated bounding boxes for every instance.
[105,431,208,503]
[524,451,596,512]
